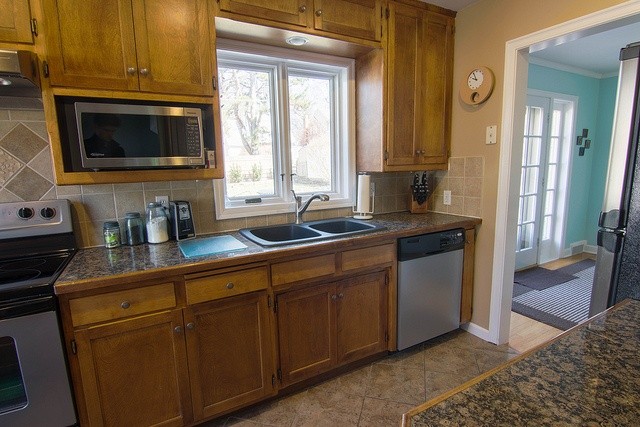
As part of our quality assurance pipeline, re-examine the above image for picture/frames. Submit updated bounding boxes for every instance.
[575,135,583,145]
[582,128,589,137]
[578,146,585,156]
[585,139,591,149]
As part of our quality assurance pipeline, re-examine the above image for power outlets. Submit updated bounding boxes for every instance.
[443,191,451,205]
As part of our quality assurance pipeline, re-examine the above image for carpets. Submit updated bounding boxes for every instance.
[515,266,578,292]
[512,258,595,331]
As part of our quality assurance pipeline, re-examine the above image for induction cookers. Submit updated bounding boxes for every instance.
[1,250,76,295]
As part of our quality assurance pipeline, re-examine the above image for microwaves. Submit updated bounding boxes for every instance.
[75,101,206,170]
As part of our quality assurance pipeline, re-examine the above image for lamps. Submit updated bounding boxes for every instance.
[286,37,308,45]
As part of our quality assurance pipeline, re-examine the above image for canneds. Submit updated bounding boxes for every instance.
[101,222,122,248]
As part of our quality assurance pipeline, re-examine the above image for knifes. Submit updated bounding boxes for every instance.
[409,169,429,190]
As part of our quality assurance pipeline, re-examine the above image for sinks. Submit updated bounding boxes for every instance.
[307,213,387,238]
[239,224,329,248]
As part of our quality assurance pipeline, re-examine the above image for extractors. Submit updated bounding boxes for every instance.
[0,48,43,97]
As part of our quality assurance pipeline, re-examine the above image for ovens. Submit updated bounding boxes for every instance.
[0,296,80,426]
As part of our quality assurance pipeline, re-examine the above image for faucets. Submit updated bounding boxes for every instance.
[290,190,329,224]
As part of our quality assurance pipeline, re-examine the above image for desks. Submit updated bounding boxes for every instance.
[399,296,640,426]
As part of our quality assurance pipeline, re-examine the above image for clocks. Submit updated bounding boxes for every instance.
[457,65,495,107]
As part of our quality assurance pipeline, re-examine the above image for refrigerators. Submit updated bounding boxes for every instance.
[588,42,639,318]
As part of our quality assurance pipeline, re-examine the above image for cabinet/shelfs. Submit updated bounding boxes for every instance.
[356,0,458,177]
[40,90,227,186]
[269,232,396,390]
[0,1,42,53]
[216,1,382,59]
[54,250,278,427]
[39,2,218,98]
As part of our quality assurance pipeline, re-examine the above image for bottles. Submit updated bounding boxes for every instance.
[125,214,144,246]
[103,221,122,249]
[145,200,170,243]
